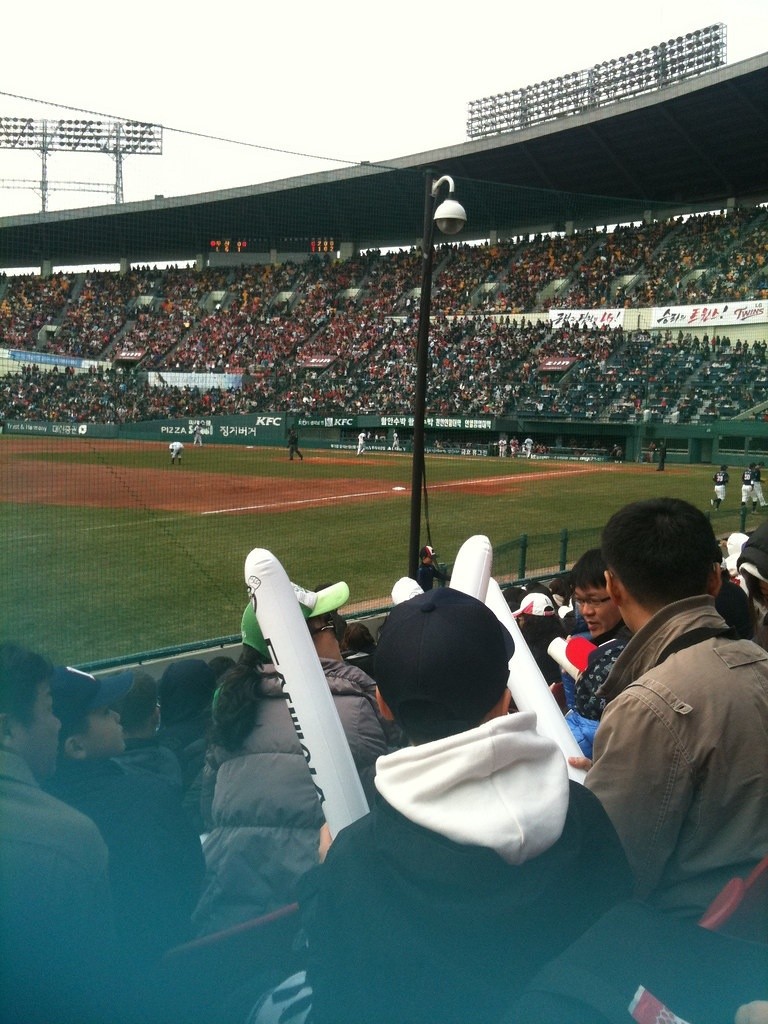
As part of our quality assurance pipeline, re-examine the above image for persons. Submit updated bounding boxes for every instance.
[612,444,622,463]
[742,462,768,513]
[711,465,729,511]
[0,497,768,1024]
[194,423,203,447]
[169,441,184,464]
[287,430,303,460]
[393,429,403,451]
[357,430,373,456]
[650,204,768,423]
[451,219,650,421]
[649,440,666,471]
[0,262,196,424]
[192,240,452,416]
[499,436,533,459]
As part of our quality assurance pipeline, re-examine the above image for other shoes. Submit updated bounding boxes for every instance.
[711,498,715,506]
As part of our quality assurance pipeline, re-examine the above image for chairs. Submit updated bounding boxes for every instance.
[698,853,768,932]
[161,903,302,1024]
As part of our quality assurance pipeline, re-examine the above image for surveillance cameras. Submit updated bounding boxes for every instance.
[433,199,467,234]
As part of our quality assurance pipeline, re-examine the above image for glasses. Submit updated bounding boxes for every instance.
[572,594,611,607]
[311,614,338,636]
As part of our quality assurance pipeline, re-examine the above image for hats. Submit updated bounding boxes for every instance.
[48,666,136,722]
[511,592,554,619]
[749,462,756,467]
[376,589,514,729]
[759,462,765,466]
[241,581,349,661]
[721,464,728,469]
[738,561,768,584]
[420,546,437,558]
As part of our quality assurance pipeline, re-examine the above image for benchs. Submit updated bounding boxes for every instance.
[516,341,768,423]
